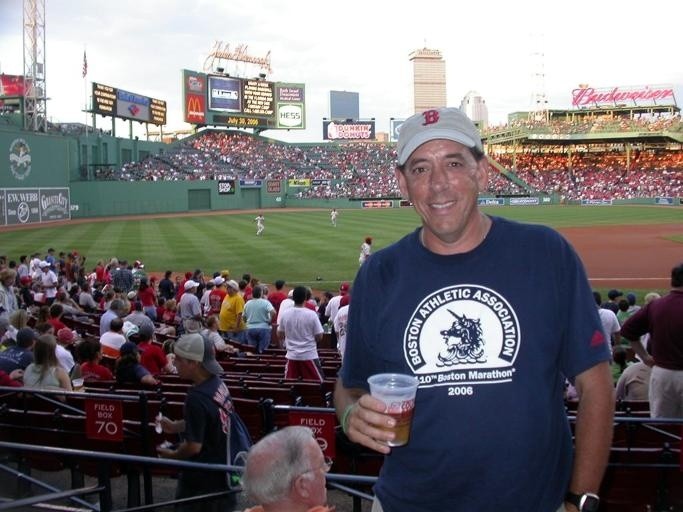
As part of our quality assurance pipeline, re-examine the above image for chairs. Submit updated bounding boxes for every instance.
[0,294,683,512]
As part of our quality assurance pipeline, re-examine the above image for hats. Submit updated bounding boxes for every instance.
[172,332,225,377]
[39,261,51,269]
[608,289,623,299]
[184,269,240,293]
[397,106,483,167]
[338,280,350,309]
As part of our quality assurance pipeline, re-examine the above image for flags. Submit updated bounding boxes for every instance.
[81,54,88,77]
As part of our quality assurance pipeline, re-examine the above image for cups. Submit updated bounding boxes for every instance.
[70,377,83,391]
[154,416,164,435]
[367,372,420,447]
